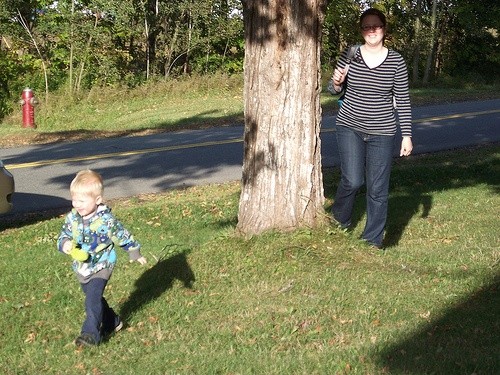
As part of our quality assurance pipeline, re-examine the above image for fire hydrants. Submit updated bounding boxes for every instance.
[20,85,39,130]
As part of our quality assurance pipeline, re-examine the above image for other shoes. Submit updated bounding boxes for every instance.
[72,335,103,351]
[100,319,123,338]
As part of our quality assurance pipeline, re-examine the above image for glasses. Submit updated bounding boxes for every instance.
[361,24,381,31]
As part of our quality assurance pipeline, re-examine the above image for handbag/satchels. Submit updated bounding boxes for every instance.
[339,45,357,108]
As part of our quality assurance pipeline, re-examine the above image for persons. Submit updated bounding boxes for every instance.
[328,8,413,254]
[56,168,148,349]
